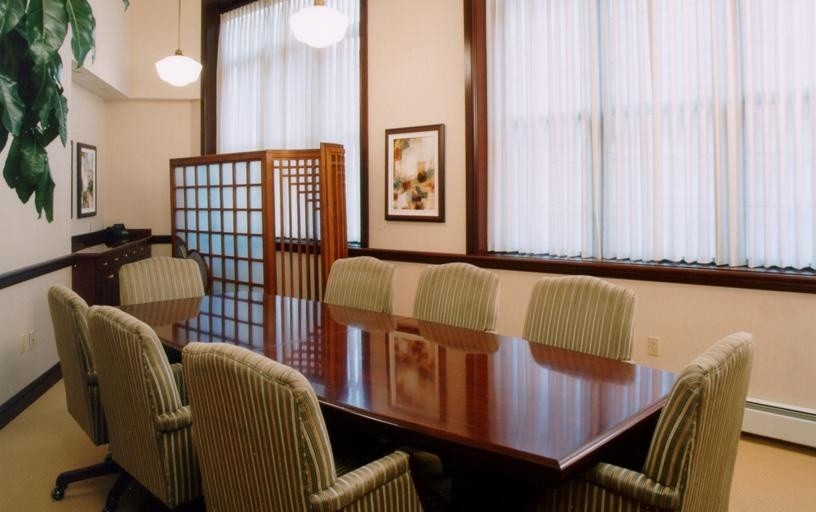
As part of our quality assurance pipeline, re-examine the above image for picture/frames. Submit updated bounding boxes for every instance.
[382,122,448,226]
[74,141,100,219]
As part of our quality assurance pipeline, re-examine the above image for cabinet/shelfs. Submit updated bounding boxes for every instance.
[66,225,152,307]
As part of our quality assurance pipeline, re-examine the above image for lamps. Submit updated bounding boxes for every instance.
[152,1,204,90]
[286,1,349,50]
